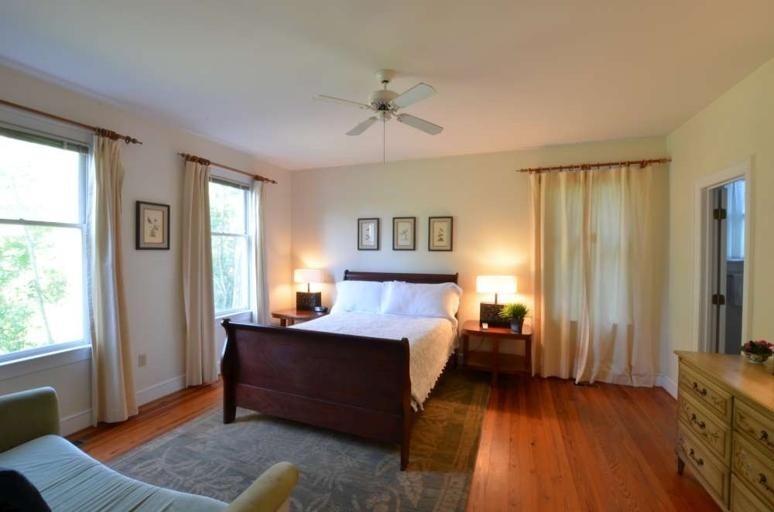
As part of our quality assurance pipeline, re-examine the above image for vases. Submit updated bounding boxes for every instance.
[747,355,767,362]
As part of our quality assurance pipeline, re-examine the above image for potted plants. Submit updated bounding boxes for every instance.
[500,305,530,333]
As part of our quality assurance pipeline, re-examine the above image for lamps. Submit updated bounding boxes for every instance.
[475,274,518,325]
[293,268,322,312]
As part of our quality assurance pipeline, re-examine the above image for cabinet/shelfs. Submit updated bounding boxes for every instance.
[672,348,773,512]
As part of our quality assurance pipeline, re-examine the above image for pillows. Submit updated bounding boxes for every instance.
[1,467,54,512]
[330,281,387,315]
[381,281,463,329]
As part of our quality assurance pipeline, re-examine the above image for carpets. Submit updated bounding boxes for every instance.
[102,370,494,511]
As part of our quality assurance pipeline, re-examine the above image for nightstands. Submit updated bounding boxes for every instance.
[461,320,534,386]
[271,307,329,327]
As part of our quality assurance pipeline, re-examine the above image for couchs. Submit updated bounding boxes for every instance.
[0,385,301,512]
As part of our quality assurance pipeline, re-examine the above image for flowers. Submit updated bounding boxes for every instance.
[740,339,773,356]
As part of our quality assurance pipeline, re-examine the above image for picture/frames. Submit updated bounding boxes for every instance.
[357,218,380,251]
[134,199,171,252]
[427,216,453,252]
[392,216,416,252]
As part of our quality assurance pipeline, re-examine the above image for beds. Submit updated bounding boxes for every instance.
[218,268,461,472]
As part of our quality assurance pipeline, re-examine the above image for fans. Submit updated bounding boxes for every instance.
[313,69,444,139]
